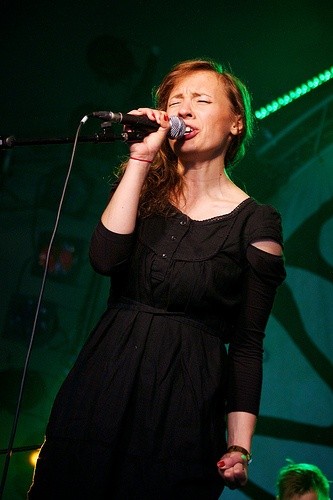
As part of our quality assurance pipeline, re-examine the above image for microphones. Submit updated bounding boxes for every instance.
[92,111,186,140]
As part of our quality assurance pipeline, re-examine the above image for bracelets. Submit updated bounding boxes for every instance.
[221,446,253,464]
[127,154,152,165]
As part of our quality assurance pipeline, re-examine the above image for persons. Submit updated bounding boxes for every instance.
[23,61,285,500]
[221,459,333,500]
